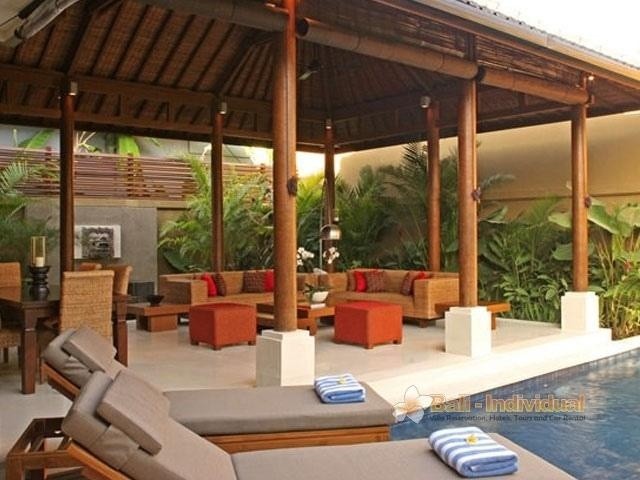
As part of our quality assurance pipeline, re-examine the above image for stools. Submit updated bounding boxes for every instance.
[332,298,404,349]
[435,299,511,330]
[189,301,256,351]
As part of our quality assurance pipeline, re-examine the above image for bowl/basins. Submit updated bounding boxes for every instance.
[150,304,160,307]
[147,294,165,304]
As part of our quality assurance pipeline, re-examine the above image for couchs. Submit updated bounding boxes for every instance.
[155,264,461,327]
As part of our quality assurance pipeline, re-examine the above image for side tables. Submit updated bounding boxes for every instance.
[126,300,188,332]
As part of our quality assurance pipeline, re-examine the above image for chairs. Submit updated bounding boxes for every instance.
[0,261,134,395]
[5,326,583,480]
